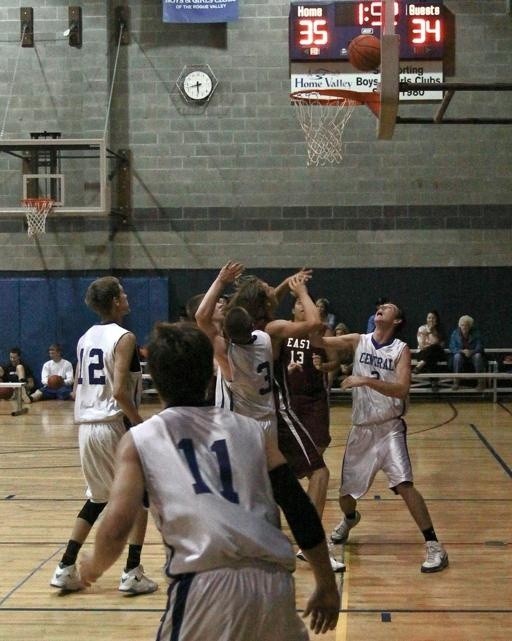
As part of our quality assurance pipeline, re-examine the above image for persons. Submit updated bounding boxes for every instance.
[448,315,488,391]
[22,344,75,405]
[46,275,160,597]
[307,302,449,575]
[184,256,353,576]
[76,320,342,641]
[365,300,387,334]
[0,348,35,401]
[411,311,447,393]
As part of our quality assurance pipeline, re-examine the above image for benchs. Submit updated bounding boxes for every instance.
[139,360,160,406]
[321,345,512,396]
[1,381,30,417]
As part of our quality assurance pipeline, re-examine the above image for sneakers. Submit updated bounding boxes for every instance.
[330,510,362,543]
[297,545,345,574]
[118,566,158,594]
[21,386,33,405]
[431,380,486,392]
[50,564,78,587]
[420,540,450,574]
[412,365,422,375]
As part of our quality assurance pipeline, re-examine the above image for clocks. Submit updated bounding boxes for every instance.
[176,64,219,105]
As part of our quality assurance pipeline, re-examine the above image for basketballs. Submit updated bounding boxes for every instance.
[48,375,63,391]
[348,35,380,72]
